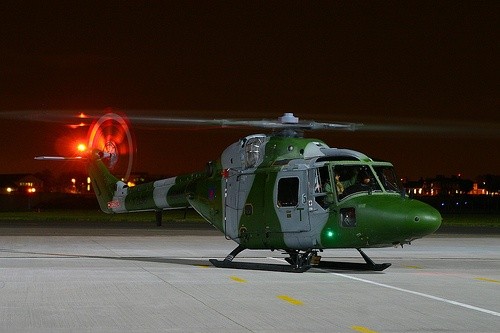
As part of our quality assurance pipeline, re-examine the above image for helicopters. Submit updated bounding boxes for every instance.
[36,115,443,272]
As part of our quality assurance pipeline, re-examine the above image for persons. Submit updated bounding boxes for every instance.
[322,168,358,207]
